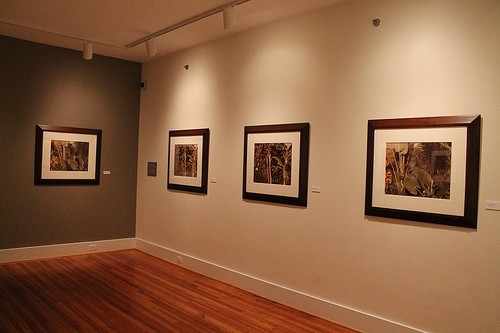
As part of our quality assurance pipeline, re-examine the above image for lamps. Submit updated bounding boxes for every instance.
[146,40,159,58]
[221,7,236,29]
[82,42,95,60]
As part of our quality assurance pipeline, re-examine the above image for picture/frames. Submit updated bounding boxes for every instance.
[242,121,311,209]
[166,128,213,195]
[364,114,482,229]
[33,124,104,185]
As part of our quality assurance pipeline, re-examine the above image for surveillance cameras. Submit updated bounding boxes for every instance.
[139,80,147,89]
